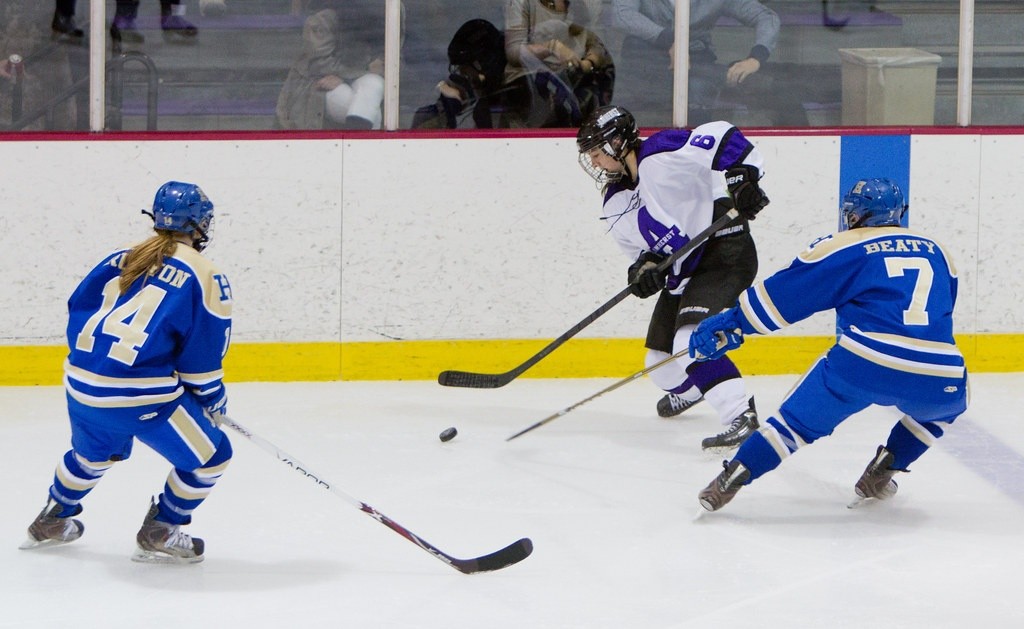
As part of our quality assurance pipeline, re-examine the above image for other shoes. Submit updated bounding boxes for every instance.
[49,19,85,47]
[109,24,145,54]
[160,15,197,45]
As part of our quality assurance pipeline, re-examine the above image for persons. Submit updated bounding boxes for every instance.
[276,0,384,131]
[577,106,770,462]
[601,0,812,128]
[48,0,198,46]
[502,0,608,129]
[688,178,969,523]
[412,17,616,129]
[17,180,236,562]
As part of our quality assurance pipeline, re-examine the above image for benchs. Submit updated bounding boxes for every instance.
[108,11,454,121]
[708,9,905,128]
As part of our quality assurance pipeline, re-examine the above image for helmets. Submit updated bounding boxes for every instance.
[577,103,639,184]
[449,14,508,87]
[840,176,911,235]
[153,182,215,253]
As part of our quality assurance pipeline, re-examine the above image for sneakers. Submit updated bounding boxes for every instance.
[17,496,84,550]
[691,459,754,523]
[129,493,205,565]
[702,394,759,461]
[656,387,704,419]
[847,444,898,509]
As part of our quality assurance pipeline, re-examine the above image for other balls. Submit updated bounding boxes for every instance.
[439,427,457,442]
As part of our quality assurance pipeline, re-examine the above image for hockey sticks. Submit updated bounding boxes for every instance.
[507,331,726,441]
[213,409,533,574]
[437,188,766,388]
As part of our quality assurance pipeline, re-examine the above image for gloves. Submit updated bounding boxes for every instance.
[627,249,674,300]
[725,162,771,222]
[211,404,230,429]
[690,306,744,361]
